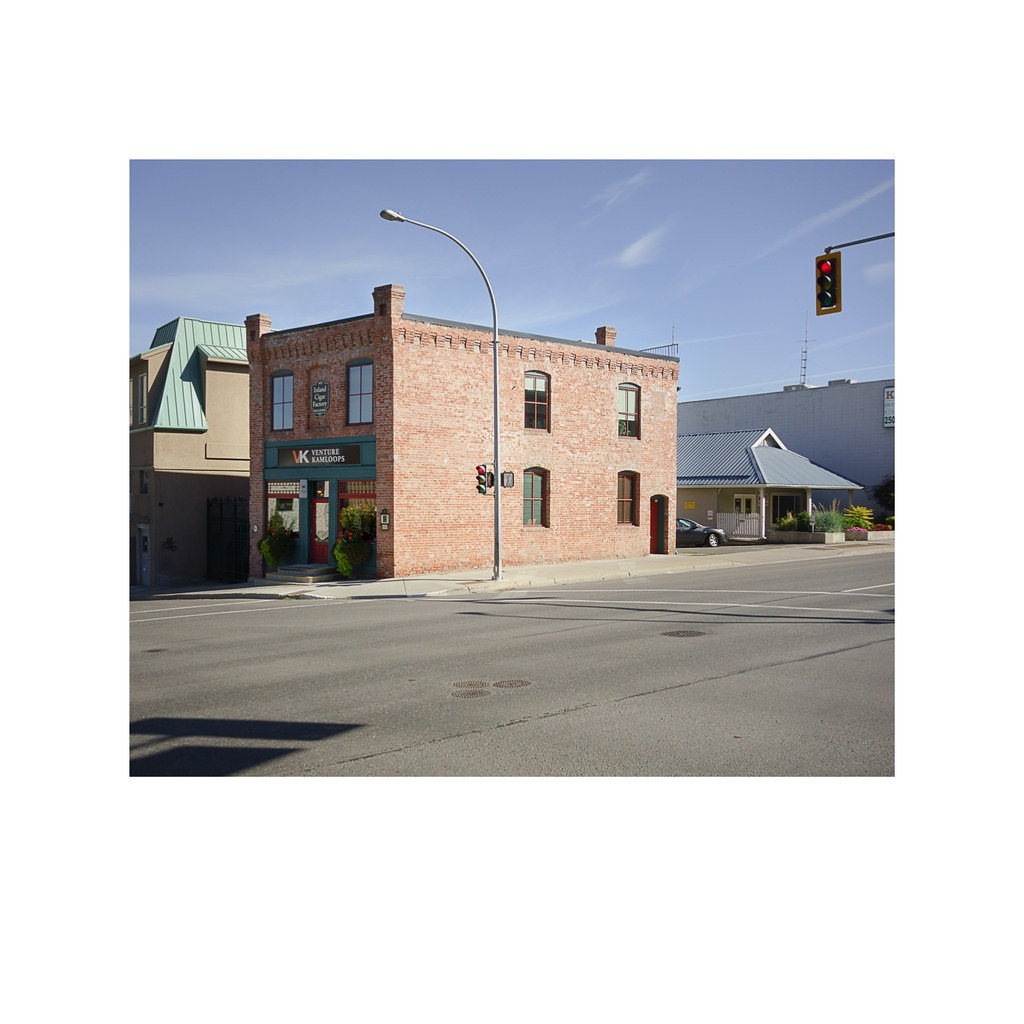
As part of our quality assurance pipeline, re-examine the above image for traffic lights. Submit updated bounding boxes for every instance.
[476,465,487,494]
[503,472,514,488]
[816,251,842,315]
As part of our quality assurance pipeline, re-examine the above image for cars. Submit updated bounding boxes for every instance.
[676,517,728,547]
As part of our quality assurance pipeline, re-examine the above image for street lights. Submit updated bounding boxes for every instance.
[380,209,501,580]
